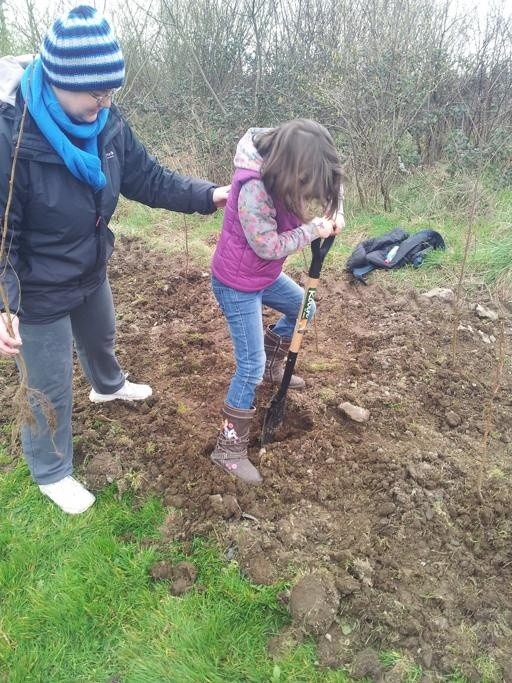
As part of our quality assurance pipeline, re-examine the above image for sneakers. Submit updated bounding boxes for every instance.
[39,475,96,515]
[89,380,153,402]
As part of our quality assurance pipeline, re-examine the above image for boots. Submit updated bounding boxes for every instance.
[210,403,264,483]
[265,324,306,388]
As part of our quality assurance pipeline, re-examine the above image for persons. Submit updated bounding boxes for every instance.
[0,7,233,514]
[211,119,346,485]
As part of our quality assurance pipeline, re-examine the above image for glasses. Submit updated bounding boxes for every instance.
[88,86,122,104]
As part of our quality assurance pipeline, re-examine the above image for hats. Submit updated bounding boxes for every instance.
[40,5,125,90]
